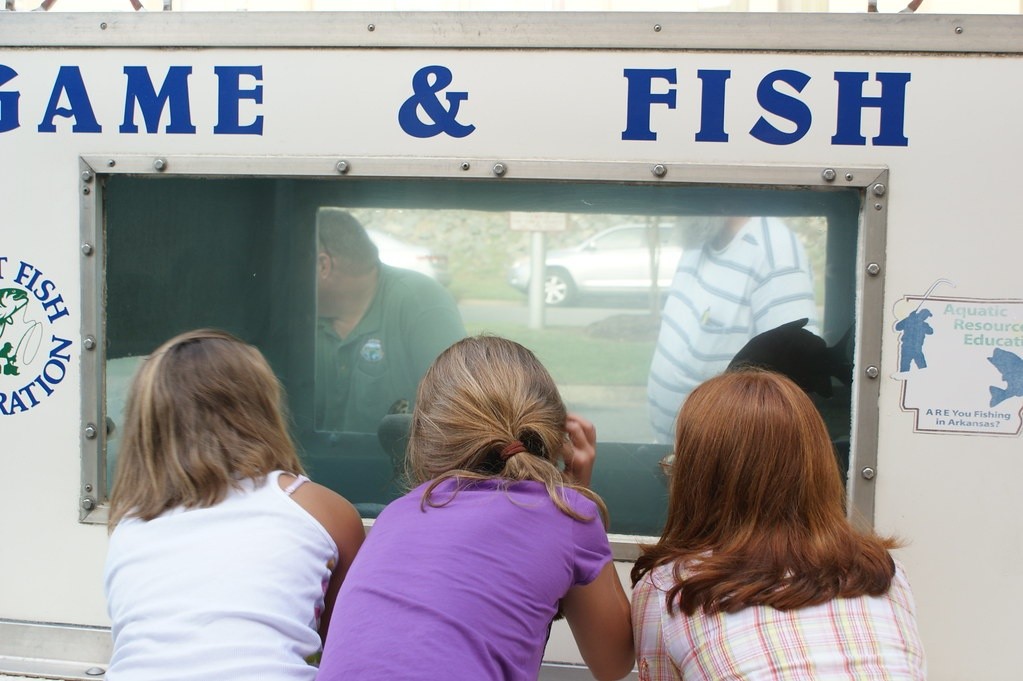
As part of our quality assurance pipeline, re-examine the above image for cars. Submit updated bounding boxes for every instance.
[366,228,450,287]
[509,223,683,307]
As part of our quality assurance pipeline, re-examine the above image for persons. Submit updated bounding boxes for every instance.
[311,333,636,681]
[104,328,365,679]
[315,208,464,433]
[629,368,925,681]
[644,214,815,447]
